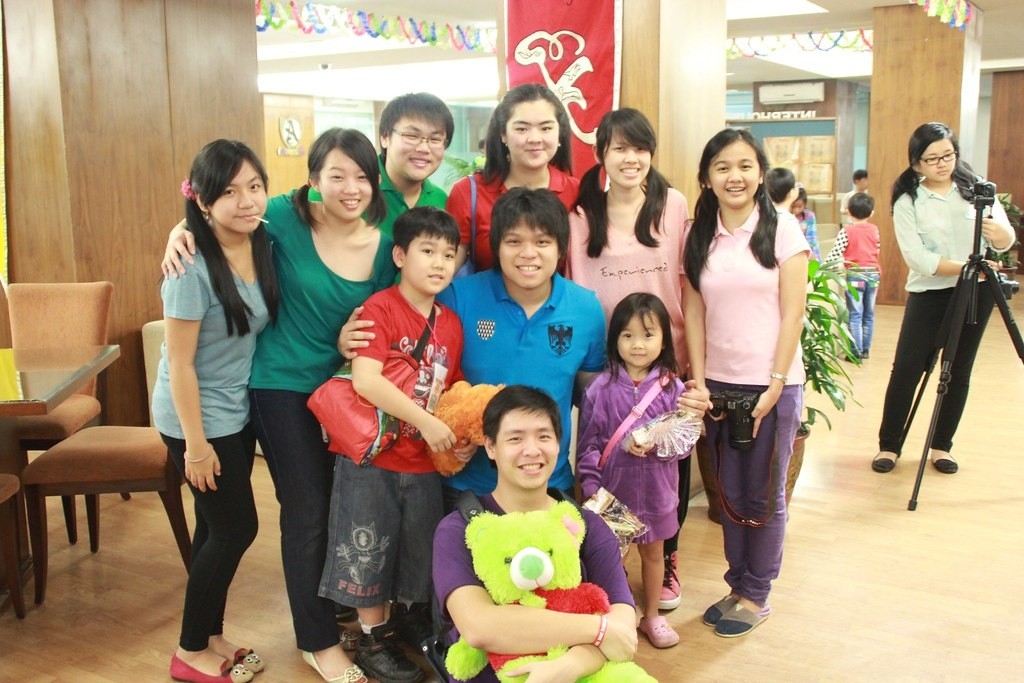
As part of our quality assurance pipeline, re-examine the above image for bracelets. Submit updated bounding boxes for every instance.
[771,372,787,386]
[183,442,213,463]
[593,615,608,647]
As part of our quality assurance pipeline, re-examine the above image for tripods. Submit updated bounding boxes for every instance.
[896,196,1024,511]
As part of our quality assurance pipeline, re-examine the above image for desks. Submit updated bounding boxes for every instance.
[0,344,121,616]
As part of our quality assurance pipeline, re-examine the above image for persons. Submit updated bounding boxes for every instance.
[557,108,693,610]
[432,384,638,683]
[359,91,447,286]
[869,120,1016,477]
[764,166,880,366]
[149,138,281,682]
[160,128,400,682]
[576,292,699,650]
[316,205,478,683]
[445,84,582,278]
[684,129,811,641]
[337,186,710,512]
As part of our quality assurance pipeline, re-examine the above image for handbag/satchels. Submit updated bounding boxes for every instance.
[305,306,437,471]
[454,174,477,278]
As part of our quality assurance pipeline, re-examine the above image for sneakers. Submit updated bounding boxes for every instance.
[657,551,683,611]
[354,622,424,683]
[391,601,433,655]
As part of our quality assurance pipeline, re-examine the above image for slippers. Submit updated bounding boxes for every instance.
[703,594,772,637]
[638,617,680,649]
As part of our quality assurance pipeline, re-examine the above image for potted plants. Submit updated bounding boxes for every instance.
[986,192,1024,281]
[697,256,878,525]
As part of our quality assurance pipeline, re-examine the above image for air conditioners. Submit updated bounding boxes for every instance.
[759,81,825,105]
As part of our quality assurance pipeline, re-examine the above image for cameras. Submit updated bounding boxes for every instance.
[709,389,760,449]
[974,182,996,197]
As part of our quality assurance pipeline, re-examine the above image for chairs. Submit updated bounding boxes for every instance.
[0,279,193,620]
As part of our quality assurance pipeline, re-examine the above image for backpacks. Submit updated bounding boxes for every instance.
[421,486,584,683]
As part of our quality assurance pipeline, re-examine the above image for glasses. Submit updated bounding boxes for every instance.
[921,150,958,166]
[393,128,449,148]
[792,183,802,188]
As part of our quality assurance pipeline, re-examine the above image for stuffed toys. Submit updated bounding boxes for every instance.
[429,380,505,477]
[444,500,660,683]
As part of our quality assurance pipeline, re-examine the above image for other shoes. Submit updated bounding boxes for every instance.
[170,647,265,683]
[335,604,353,618]
[302,624,368,683]
[872,449,901,472]
[933,454,958,473]
[845,350,869,362]
